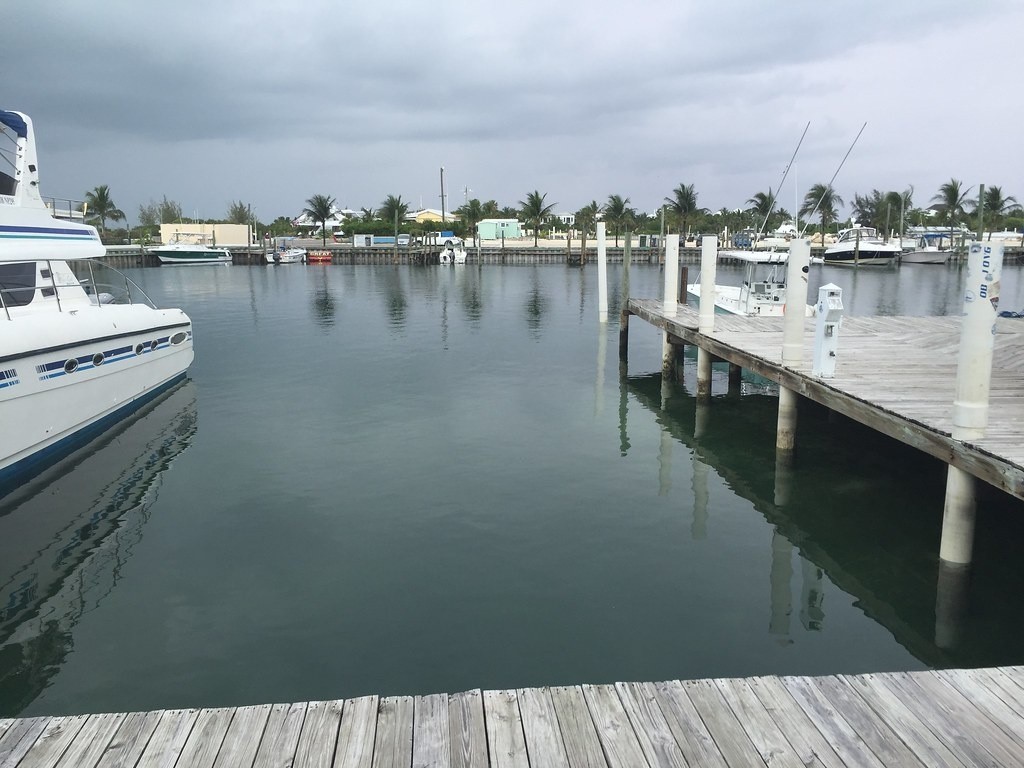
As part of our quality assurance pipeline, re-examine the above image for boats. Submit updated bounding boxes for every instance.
[264,247,307,264]
[895,232,957,265]
[686,118,865,316]
[438,245,467,265]
[308,249,332,263]
[822,217,904,267]
[0,109,197,483]
[144,229,233,263]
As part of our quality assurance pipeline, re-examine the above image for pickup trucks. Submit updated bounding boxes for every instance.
[413,232,462,247]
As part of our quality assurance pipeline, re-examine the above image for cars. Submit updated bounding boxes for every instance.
[679,235,685,247]
[733,232,753,247]
[695,233,721,247]
[398,233,410,245]
